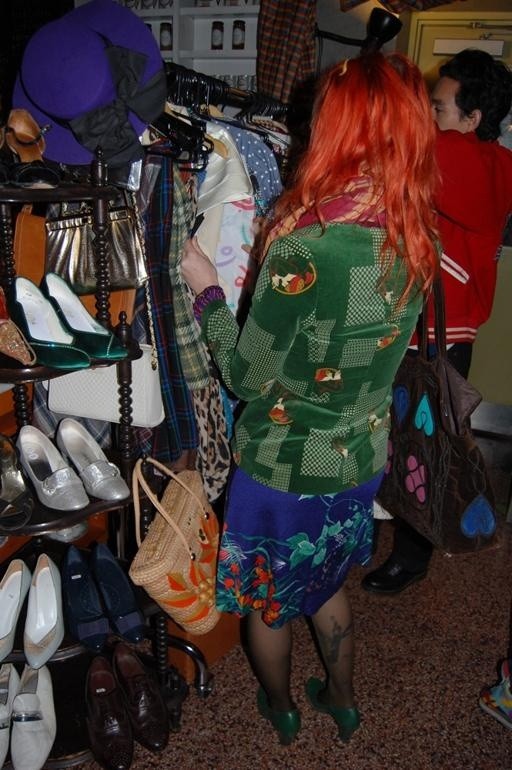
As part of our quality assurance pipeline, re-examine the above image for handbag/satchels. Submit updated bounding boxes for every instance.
[129,470,222,636]
[11,207,147,326]
[376,352,504,559]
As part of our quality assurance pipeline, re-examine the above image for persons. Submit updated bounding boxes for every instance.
[178,51,445,746]
[360,47,512,596]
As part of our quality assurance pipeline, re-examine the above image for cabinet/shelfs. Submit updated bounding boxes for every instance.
[1,145,211,768]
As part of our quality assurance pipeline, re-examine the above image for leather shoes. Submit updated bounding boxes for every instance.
[6,109,61,190]
[362,561,427,596]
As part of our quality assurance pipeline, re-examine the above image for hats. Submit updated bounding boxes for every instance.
[12,1,168,166]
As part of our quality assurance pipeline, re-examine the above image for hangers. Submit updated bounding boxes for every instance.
[145,56,298,173]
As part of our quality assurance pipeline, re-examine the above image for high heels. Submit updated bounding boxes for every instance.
[306,677,360,744]
[257,685,301,745]
